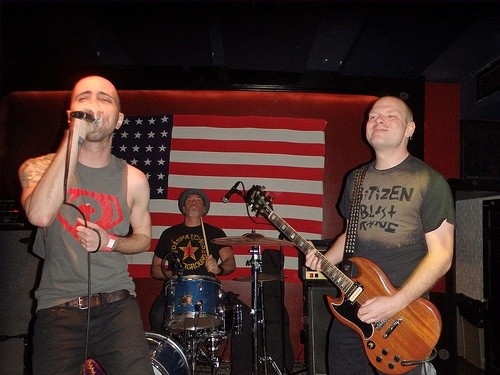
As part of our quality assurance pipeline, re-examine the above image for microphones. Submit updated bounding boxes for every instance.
[172,252,183,269]
[72,111,99,124]
[222,181,241,203]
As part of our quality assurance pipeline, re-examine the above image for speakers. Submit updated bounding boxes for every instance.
[302,283,341,375]
[0,222,45,375]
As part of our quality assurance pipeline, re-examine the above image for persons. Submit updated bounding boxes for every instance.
[17,76,151,375]
[305,96,455,375]
[151,189,255,375]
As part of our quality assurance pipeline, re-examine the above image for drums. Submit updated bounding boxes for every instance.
[164,274,226,331]
[144,331,191,375]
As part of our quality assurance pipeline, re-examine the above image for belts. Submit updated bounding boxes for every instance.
[49,289,130,310]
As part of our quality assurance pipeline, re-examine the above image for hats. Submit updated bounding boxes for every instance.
[178,189,210,217]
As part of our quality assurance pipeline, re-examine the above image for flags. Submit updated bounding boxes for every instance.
[111,115,326,283]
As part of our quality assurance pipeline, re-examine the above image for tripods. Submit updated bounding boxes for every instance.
[258,285,283,375]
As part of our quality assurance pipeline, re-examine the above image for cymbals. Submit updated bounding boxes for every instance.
[209,232,297,246]
[231,275,289,282]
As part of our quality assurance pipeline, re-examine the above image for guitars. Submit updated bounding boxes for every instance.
[242,184,445,375]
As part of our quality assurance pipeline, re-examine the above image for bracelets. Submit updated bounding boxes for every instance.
[105,231,117,252]
[217,264,224,276]
